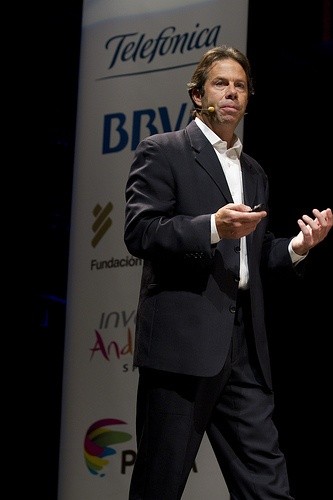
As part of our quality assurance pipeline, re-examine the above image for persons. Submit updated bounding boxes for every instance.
[123,45,332,500]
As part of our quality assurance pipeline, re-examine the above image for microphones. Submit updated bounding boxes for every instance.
[195,105,215,113]
